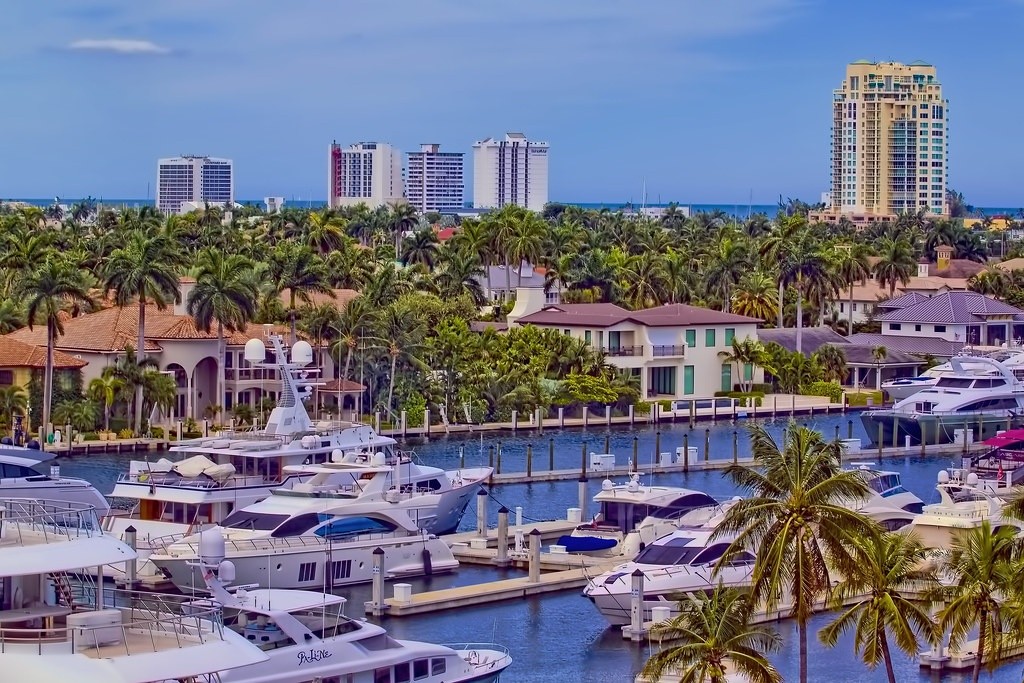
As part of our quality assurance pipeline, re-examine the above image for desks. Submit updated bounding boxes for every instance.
[0,607,72,636]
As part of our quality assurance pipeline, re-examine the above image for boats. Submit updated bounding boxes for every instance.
[148,453,459,596]
[580,495,760,629]
[2,328,493,576]
[881,343,1024,402]
[865,356,1024,444]
[754,463,927,533]
[0,496,514,683]
[556,474,744,559]
[886,468,1024,603]
[947,428,1024,488]
[0,442,112,523]
